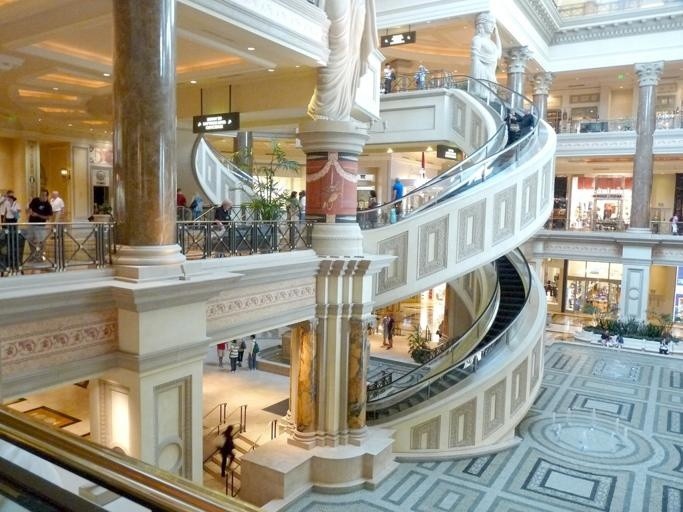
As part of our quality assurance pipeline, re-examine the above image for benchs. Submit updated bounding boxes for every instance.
[1,254,97,272]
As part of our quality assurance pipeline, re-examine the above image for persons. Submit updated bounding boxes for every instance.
[672,211,679,235]
[601,330,624,349]
[504,108,535,144]
[217,343,225,367]
[248,335,256,370]
[229,338,247,371]
[384,63,395,93]
[26,191,64,239]
[0,191,22,230]
[383,202,388,225]
[287,191,306,230]
[369,190,378,228]
[413,60,430,90]
[382,313,395,349]
[391,178,403,208]
[659,337,668,354]
[25,189,53,262]
[217,426,235,477]
[214,200,232,230]
[177,189,204,230]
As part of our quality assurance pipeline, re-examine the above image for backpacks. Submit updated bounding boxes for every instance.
[251,340,259,353]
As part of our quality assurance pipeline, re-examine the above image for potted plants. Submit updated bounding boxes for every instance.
[87,203,115,225]
[575,311,683,355]
[229,143,301,248]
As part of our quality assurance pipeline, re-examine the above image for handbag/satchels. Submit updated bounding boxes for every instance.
[214,434,227,449]
[241,341,246,348]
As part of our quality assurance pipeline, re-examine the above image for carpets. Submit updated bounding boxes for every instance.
[263,396,290,417]
